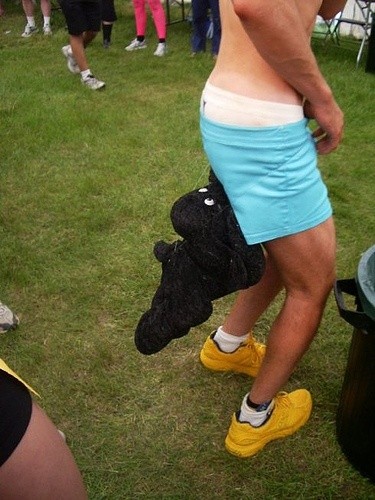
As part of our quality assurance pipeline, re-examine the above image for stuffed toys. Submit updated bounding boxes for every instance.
[134,167,265,355]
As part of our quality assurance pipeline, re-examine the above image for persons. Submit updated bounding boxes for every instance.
[99,0,116,50]
[125,0,168,56]
[0,301,19,334]
[22,0,53,37]
[57,0,105,91]
[189,0,223,59]
[0,358,89,500]
[199,0,347,460]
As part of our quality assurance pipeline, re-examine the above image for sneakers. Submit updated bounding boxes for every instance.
[80,74,108,90]
[199,330,268,377]
[225,388,312,458]
[62,44,81,74]
[42,23,52,36]
[125,37,147,51]
[21,23,39,37]
[153,42,169,57]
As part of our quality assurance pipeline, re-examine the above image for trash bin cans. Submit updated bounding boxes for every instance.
[333,245,374,484]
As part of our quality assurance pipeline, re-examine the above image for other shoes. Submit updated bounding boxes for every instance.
[0,301,21,334]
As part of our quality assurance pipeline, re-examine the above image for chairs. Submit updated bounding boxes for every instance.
[309,0,375,68]
